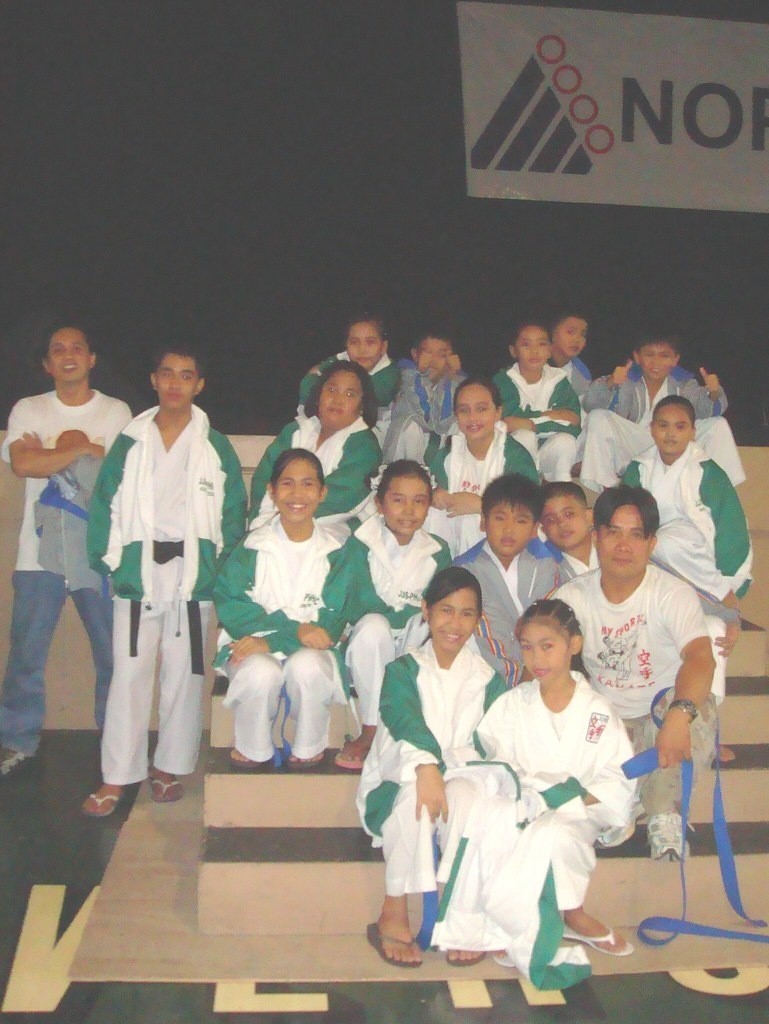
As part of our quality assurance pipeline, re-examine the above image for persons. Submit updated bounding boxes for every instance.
[214,310,751,858]
[0,325,135,774]
[465,599,638,962]
[78,339,248,820]
[358,566,510,965]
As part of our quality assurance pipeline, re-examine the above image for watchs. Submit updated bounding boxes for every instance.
[668,699,698,724]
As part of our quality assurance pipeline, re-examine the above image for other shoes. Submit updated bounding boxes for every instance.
[287,752,325,767]
[0,746,24,774]
[230,747,263,769]
[335,740,369,770]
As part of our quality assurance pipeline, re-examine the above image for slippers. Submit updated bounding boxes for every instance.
[562,920,634,956]
[493,949,515,967]
[148,768,182,802]
[444,949,486,968]
[84,786,126,817]
[366,922,423,969]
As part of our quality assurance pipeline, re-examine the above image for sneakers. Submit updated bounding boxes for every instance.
[646,812,694,865]
[594,801,647,851]
